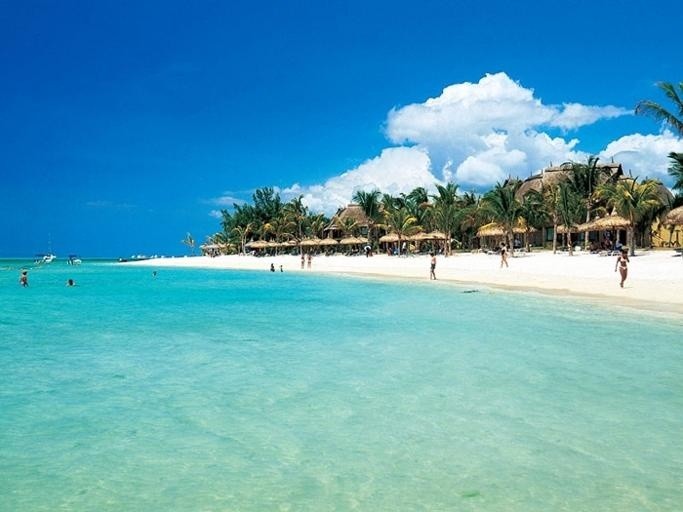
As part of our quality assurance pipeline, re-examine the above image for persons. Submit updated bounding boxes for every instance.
[301,254,313,268]
[280,265,283,272]
[270,263,275,272]
[429,253,437,281]
[500,243,508,269]
[589,235,623,254]
[208,248,215,259]
[615,248,629,288]
[391,238,406,254]
[364,246,372,258]
[68,279,73,286]
[21,270,29,288]
[514,238,521,246]
